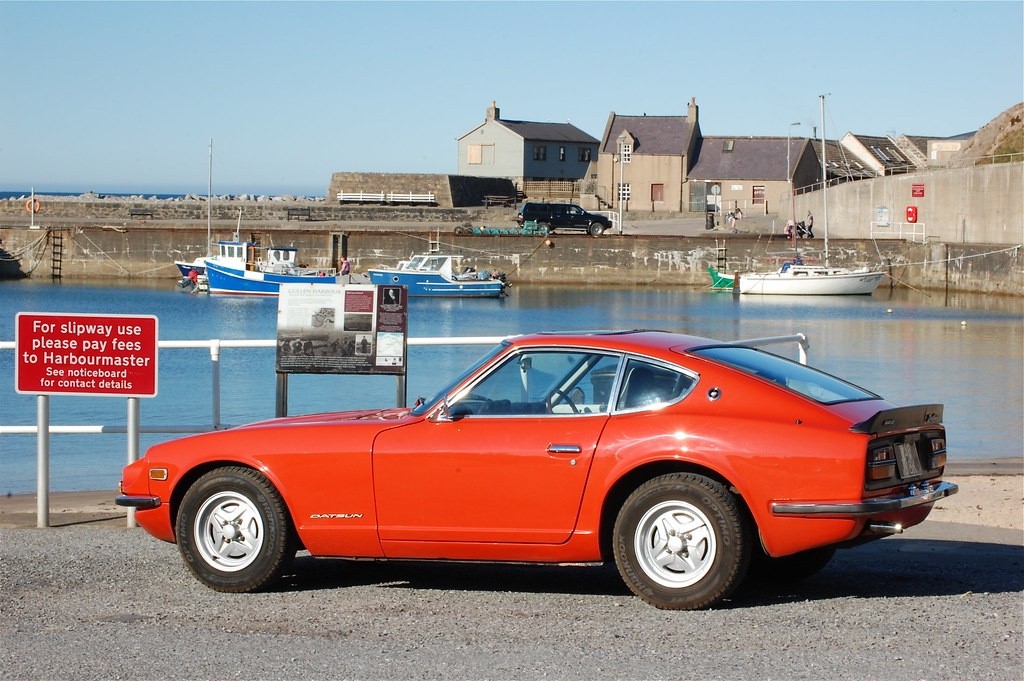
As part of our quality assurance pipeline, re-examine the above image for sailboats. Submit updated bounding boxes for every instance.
[175,139,213,290]
[738,93,887,296]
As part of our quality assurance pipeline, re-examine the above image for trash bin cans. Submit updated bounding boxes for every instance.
[706,213,714,229]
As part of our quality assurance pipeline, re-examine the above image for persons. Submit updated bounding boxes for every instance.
[336,255,350,276]
[303,339,315,355]
[281,339,291,352]
[360,336,368,353]
[292,339,302,355]
[787,219,794,233]
[726,208,742,229]
[333,337,355,356]
[806,210,814,239]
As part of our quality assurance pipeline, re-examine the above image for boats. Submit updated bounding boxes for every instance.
[366,251,512,299]
[204,210,351,296]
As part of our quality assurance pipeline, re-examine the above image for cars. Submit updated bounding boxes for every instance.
[115,331,957,611]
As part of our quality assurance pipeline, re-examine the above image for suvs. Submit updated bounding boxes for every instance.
[518,202,612,236]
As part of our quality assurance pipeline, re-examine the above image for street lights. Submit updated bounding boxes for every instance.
[619,136,627,234]
[787,122,802,251]
